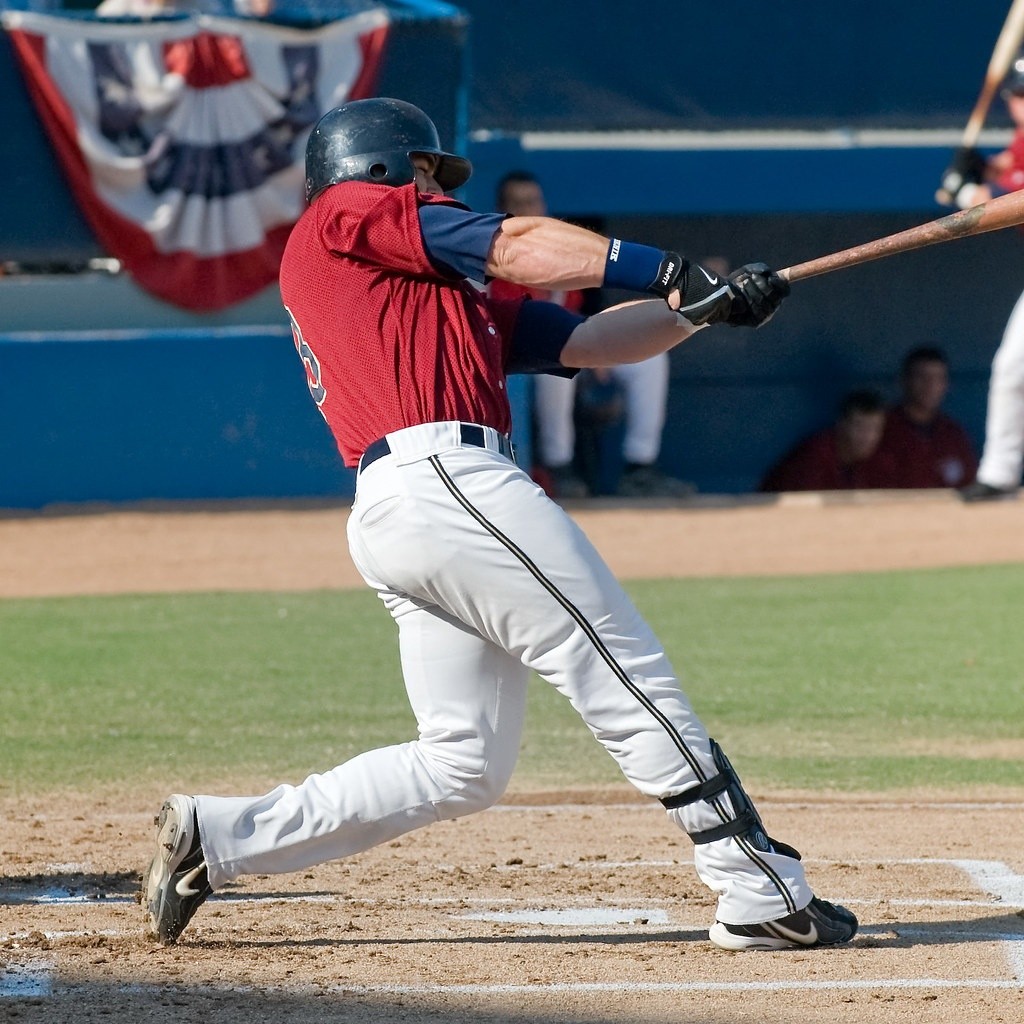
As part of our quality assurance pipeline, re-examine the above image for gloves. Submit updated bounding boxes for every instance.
[724,260,794,330]
[646,248,739,327]
[950,142,987,186]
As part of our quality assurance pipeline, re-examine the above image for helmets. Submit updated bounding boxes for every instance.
[302,96,474,205]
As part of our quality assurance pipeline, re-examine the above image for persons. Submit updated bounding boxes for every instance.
[136,96,860,950]
[946,58,1024,504]
[481,164,668,508]
[757,339,979,490]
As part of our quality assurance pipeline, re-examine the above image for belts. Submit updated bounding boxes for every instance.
[359,422,517,475]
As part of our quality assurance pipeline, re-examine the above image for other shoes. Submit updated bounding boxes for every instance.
[615,442,698,496]
[550,465,592,500]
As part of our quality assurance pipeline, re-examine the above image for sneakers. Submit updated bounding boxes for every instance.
[707,892,860,952]
[132,793,216,948]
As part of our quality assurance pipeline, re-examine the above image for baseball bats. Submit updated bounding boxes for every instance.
[933,3,1024,209]
[679,184,1024,301]
